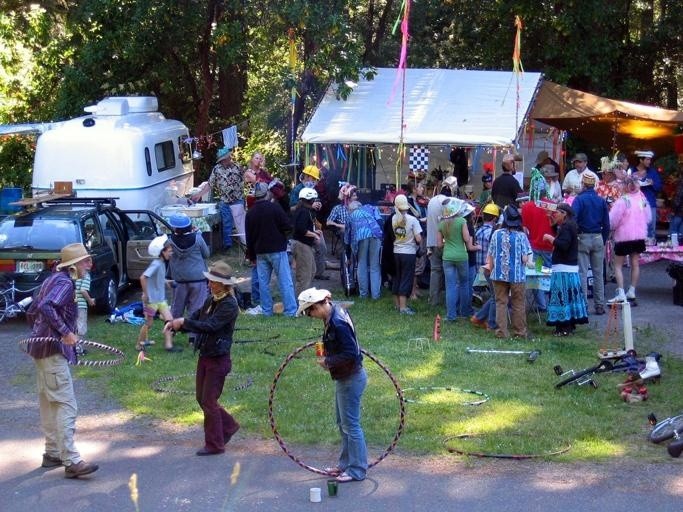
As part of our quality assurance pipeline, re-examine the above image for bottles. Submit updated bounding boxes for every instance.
[535,254,543,273]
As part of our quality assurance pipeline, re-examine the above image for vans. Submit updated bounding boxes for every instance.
[31,95,195,228]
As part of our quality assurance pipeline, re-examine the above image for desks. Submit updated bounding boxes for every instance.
[473,264,552,327]
[156,210,222,256]
[605,240,683,267]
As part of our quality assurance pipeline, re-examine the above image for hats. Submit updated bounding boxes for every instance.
[252,182,268,197]
[395,194,410,211]
[441,197,522,226]
[556,202,575,216]
[202,260,236,285]
[482,173,492,182]
[295,287,331,318]
[535,151,595,184]
[634,150,654,158]
[215,148,233,163]
[56,243,97,271]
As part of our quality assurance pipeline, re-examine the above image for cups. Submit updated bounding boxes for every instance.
[316,343,324,361]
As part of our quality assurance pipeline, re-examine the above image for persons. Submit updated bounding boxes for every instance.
[26,244,97,477]
[162,262,238,456]
[183,146,433,317]
[74,255,95,355]
[135,234,179,352]
[294,287,368,483]
[426,148,683,341]
[165,215,210,342]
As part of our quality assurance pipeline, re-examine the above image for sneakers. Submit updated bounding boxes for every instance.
[43,454,62,467]
[65,459,98,478]
[399,307,416,315]
[595,305,606,314]
[324,468,353,482]
[246,305,265,315]
[167,346,183,351]
[470,315,576,337]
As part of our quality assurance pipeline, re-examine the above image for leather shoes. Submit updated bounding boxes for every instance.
[224,425,240,446]
[196,447,225,455]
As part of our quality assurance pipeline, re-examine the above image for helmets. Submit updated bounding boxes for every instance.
[148,233,169,258]
[168,211,192,228]
[298,165,320,200]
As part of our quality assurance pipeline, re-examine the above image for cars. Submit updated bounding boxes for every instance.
[0,195,178,312]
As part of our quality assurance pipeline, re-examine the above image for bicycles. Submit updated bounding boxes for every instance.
[646,410,682,457]
[0,262,77,324]
[551,349,662,401]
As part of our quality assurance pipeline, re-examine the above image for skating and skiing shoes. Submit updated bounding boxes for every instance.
[639,357,660,384]
[606,286,638,309]
